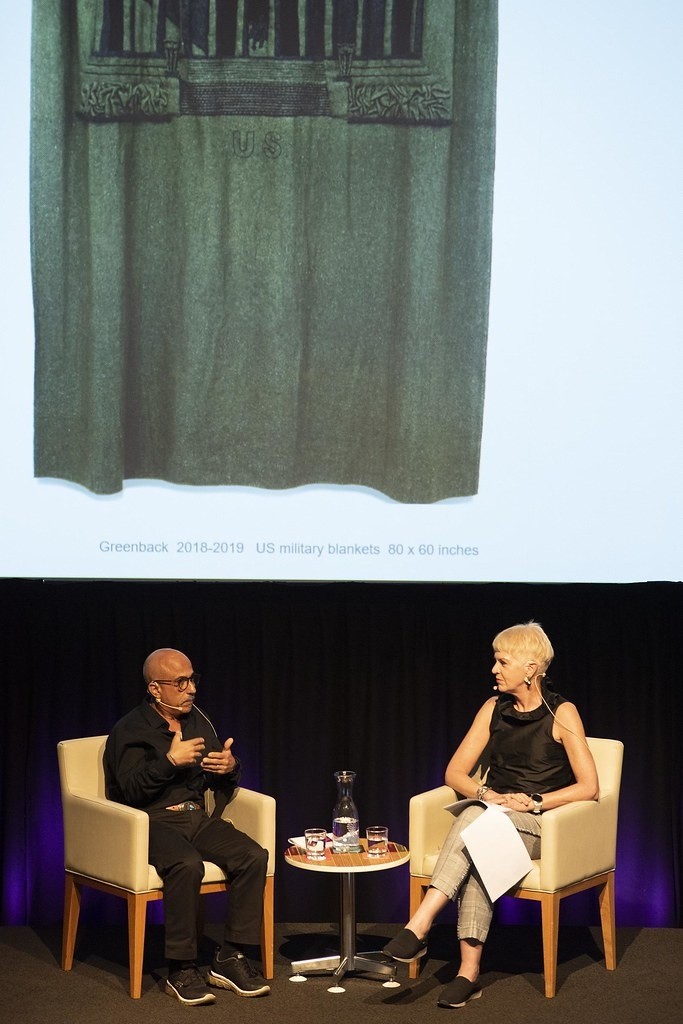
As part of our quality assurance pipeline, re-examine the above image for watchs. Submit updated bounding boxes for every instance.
[530,793,544,813]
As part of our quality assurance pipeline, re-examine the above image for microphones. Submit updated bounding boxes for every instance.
[493,686,498,690]
[160,701,183,711]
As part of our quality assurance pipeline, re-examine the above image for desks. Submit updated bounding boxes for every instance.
[284,838,410,993]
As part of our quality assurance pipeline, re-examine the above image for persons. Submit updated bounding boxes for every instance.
[379,623,600,1008]
[104,647,271,1007]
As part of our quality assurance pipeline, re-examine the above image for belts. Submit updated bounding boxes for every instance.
[164,801,205,813]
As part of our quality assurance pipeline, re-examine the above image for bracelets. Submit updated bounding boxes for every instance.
[476,786,492,800]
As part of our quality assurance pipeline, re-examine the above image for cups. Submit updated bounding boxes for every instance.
[366,826,388,856]
[305,828,326,860]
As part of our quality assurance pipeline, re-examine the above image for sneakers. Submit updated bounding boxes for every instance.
[206,951,272,997]
[165,964,215,1005]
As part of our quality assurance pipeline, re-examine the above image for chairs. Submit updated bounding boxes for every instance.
[57,735,276,999]
[409,736,624,1000]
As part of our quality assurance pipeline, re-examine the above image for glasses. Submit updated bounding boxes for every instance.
[152,672,201,690]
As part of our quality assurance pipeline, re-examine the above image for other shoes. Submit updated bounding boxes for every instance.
[382,929,428,963]
[437,976,483,1008]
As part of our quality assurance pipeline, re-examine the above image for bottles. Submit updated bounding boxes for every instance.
[332,770,360,853]
